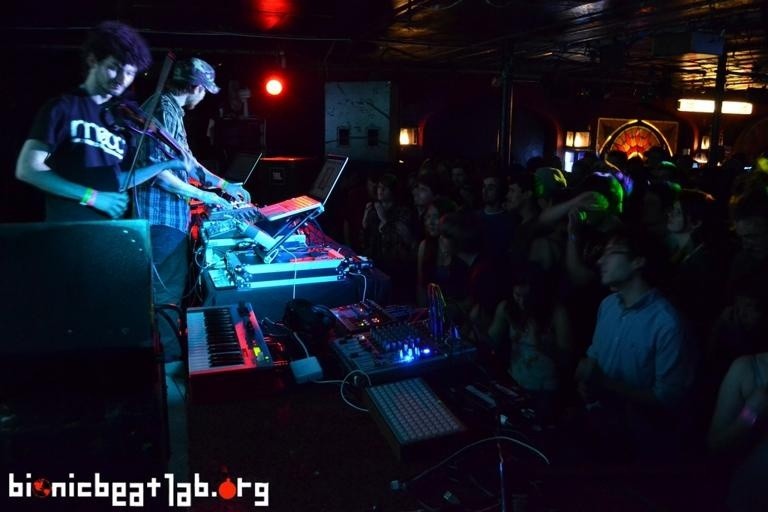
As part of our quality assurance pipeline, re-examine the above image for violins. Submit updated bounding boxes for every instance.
[111,100,184,159]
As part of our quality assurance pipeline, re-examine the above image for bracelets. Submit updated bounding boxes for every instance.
[78,186,94,207]
[217,178,225,189]
[88,190,97,207]
[221,181,229,191]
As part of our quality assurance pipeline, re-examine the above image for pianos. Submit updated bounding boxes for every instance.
[186,303,274,382]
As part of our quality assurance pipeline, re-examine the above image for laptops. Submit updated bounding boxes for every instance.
[258,155,348,223]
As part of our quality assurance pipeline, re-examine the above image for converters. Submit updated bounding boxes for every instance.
[288,355,323,385]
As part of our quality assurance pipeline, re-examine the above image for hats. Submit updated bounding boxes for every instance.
[174,56,220,95]
[534,167,568,196]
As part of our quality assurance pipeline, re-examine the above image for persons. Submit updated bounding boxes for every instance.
[15,19,200,222]
[128,54,254,364]
